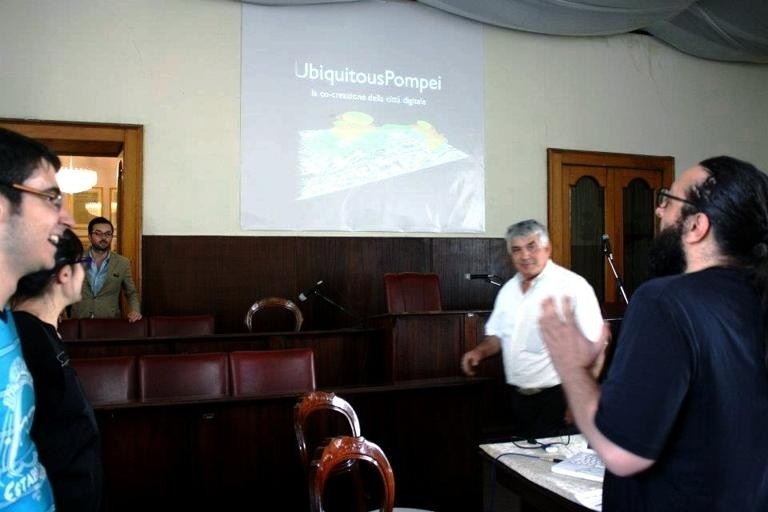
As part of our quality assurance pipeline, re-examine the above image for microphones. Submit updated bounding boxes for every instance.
[603,233,613,260]
[465,272,496,280]
[299,277,324,301]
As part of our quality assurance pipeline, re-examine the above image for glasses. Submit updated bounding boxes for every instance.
[92,230,113,236]
[656,188,694,207]
[12,182,62,208]
[71,256,93,269]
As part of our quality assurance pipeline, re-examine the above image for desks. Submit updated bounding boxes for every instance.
[479,434,600,511]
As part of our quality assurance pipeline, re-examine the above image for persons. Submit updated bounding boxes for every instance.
[58,217,142,323]
[9,228,108,512]
[537,156,768,512]
[0,128,75,512]
[460,220,608,512]
